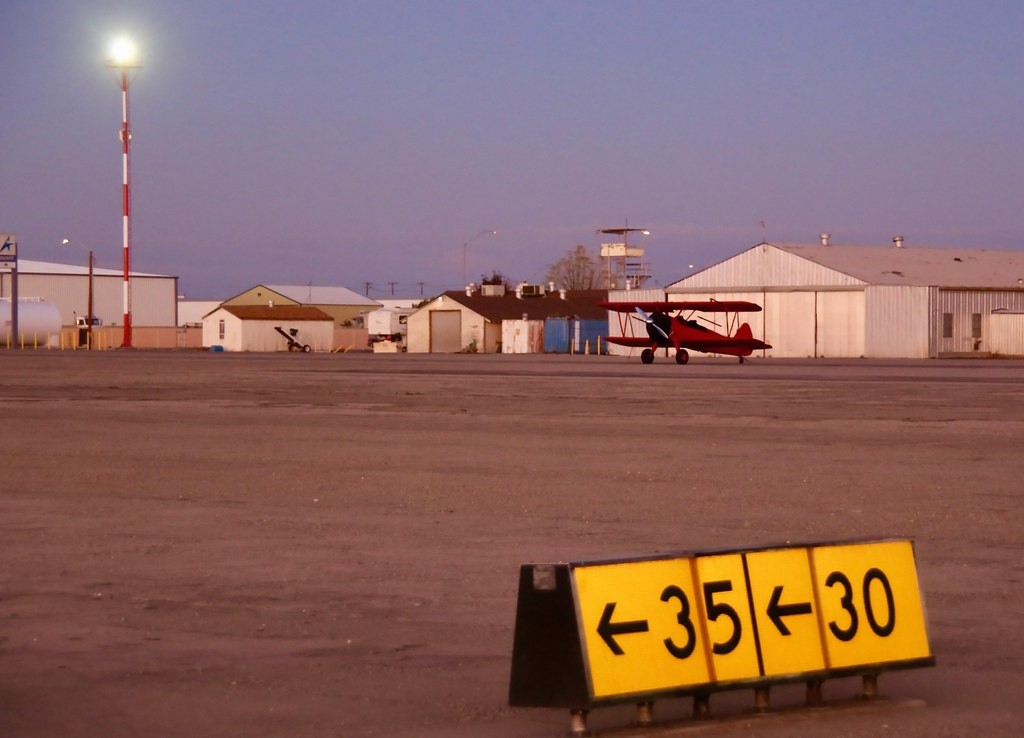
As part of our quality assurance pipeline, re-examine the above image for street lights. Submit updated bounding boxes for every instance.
[462,230,497,288]
[608,230,650,291]
[105,33,142,350]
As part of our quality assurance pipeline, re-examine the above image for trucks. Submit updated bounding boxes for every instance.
[76,315,102,329]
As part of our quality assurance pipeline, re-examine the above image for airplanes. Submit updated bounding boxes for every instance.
[598,298,775,365]
